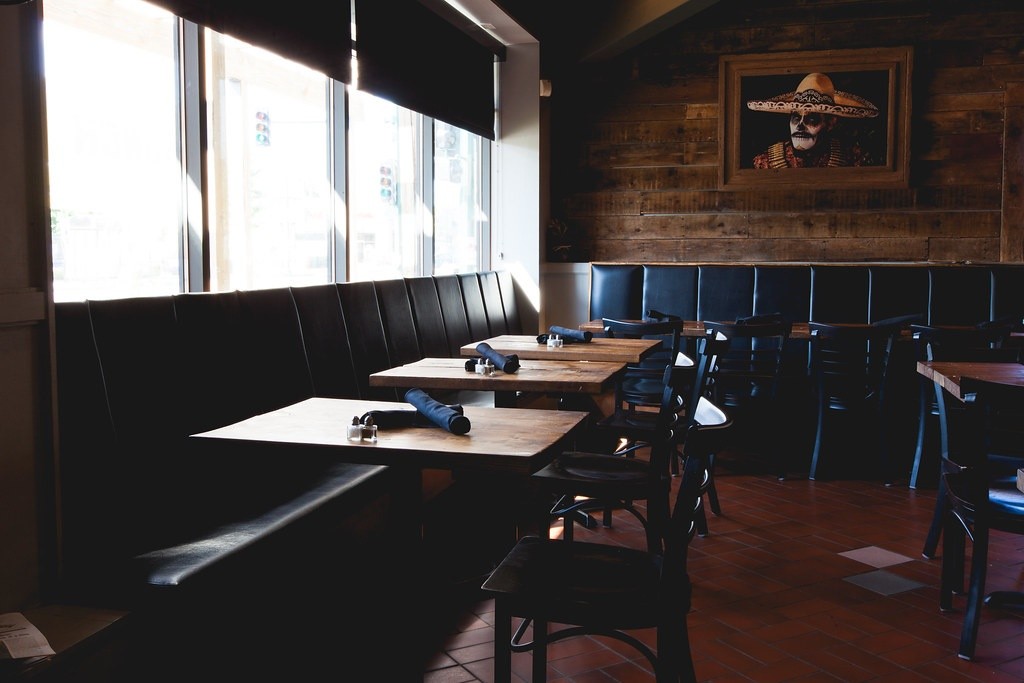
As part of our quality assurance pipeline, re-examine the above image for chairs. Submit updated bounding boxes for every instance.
[472,310,1024,683]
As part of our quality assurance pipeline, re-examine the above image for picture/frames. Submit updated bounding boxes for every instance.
[717,44,915,191]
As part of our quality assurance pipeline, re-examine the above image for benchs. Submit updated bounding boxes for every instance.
[55,273,521,602]
[588,262,1024,382]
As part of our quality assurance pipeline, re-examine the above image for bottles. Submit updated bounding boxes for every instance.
[553,334,563,347]
[475,358,485,373]
[346,416,364,441]
[361,415,377,442]
[483,358,494,375]
[547,334,554,346]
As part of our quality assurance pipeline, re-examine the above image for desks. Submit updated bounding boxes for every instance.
[461,334,664,526]
[368,356,627,539]
[188,397,591,682]
[583,314,1024,480]
[915,359,1024,590]
